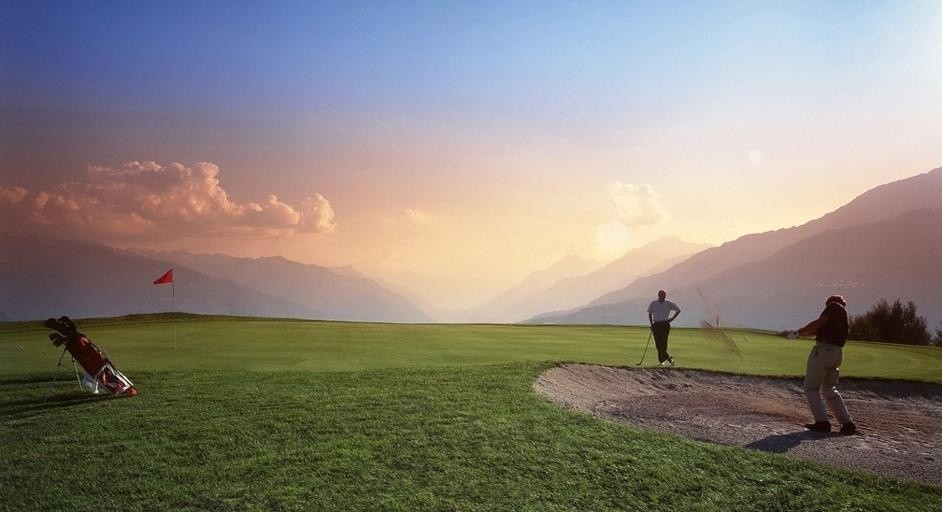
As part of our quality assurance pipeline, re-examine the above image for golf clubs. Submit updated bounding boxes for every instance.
[636,331,653,365]
[702,320,789,337]
[45,316,79,347]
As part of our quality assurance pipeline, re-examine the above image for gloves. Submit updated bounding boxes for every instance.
[784,330,799,339]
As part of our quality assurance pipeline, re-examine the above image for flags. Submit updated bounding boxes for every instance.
[152,268,173,285]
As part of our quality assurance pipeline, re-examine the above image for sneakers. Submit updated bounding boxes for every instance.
[805,421,831,433]
[841,421,857,433]
[657,358,675,367]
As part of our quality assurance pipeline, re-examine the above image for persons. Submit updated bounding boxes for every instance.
[785,295,856,434]
[647,289,681,366]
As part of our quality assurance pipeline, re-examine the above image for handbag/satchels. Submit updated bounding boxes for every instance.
[66,333,139,397]
